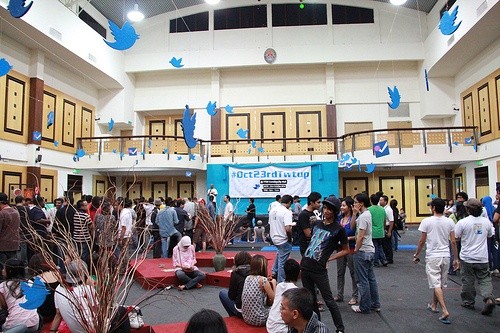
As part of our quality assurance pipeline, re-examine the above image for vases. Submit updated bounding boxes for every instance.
[213,252,226,272]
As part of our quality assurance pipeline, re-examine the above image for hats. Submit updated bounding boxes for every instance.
[153,199,163,205]
[65,259,88,284]
[322,196,341,214]
[462,198,482,209]
[427,199,446,208]
[257,220,262,225]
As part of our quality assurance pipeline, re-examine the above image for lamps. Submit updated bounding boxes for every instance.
[127,0,144,21]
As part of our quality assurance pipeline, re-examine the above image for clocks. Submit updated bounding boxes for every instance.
[264,48,277,63]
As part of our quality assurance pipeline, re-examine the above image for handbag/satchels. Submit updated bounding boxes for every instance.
[260,277,274,306]
[119,305,144,329]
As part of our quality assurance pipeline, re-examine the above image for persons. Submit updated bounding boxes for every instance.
[413,198,460,324]
[0,184,233,333]
[219,191,407,333]
[444,182,500,313]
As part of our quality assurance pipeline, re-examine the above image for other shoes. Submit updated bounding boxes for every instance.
[317,298,327,312]
[490,268,499,275]
[375,247,399,267]
[336,330,344,333]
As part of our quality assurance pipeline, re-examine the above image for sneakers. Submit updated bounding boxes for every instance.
[351,305,362,313]
[461,303,474,310]
[194,283,202,288]
[178,285,183,291]
[372,308,381,312]
[481,296,497,316]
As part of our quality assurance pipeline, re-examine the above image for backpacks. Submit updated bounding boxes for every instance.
[393,208,403,238]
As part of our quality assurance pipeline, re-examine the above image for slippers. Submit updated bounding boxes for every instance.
[427,304,439,313]
[438,315,451,324]
[348,297,358,305]
[334,295,343,302]
